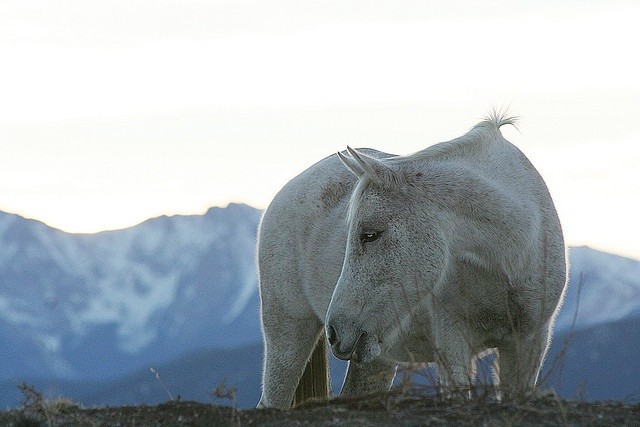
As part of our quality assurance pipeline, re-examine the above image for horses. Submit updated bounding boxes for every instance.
[257,107,569,409]
